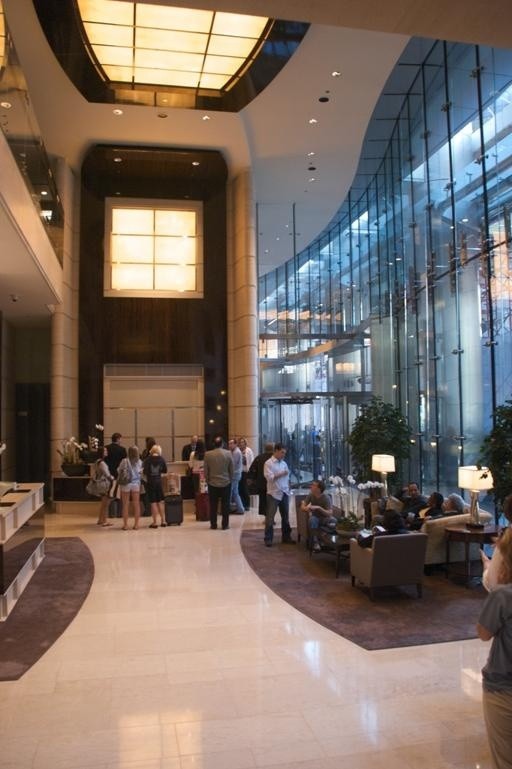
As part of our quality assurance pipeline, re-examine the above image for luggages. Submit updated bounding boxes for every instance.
[163,493,184,525]
[180,473,200,500]
[195,491,211,522]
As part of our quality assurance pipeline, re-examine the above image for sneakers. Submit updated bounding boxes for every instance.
[312,543,321,553]
[148,524,158,529]
[161,522,168,527]
[264,538,273,547]
[280,535,296,545]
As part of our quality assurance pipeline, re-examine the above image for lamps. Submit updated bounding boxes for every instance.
[457,465,494,533]
[371,455,397,498]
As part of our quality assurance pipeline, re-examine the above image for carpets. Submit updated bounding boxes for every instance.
[238,508,495,652]
[0,536,97,682]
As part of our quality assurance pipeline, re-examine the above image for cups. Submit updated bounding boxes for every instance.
[407,513,415,518]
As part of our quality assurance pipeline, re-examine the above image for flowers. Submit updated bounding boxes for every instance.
[57,436,90,465]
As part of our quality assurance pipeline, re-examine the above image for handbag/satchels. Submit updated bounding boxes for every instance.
[108,478,122,501]
[140,484,146,495]
[118,459,129,485]
[85,459,111,497]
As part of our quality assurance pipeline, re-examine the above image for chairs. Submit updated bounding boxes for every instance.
[294,486,502,605]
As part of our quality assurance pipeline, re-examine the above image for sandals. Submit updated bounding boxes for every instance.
[96,519,108,525]
[102,522,114,527]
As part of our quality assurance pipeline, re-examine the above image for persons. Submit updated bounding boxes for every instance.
[355,480,464,550]
[248,443,298,546]
[477,536,511,768]
[301,479,337,550]
[93,433,254,530]
[479,492,511,589]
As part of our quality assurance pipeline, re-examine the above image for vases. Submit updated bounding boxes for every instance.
[61,462,86,477]
